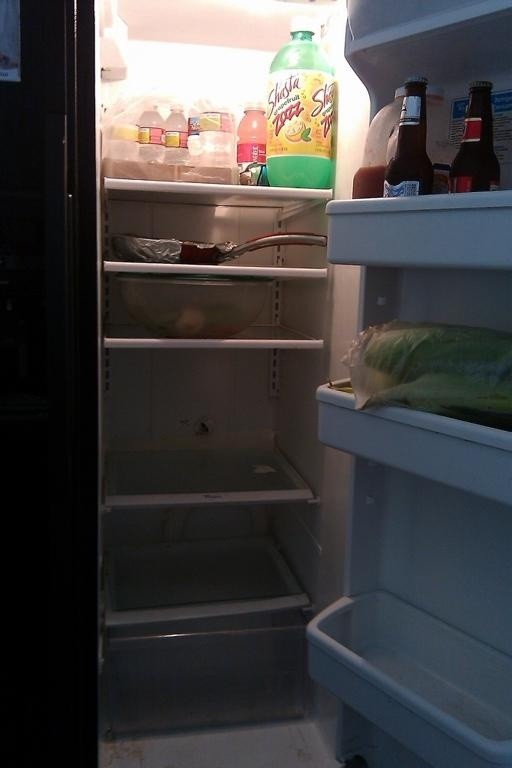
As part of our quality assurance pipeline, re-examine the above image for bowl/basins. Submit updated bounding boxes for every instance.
[111,275,272,340]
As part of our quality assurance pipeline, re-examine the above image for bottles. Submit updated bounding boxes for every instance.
[382,74,433,197]
[238,104,268,187]
[263,16,334,188]
[448,79,500,192]
[101,95,236,183]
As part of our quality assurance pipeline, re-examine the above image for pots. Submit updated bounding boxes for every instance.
[111,230,328,266]
